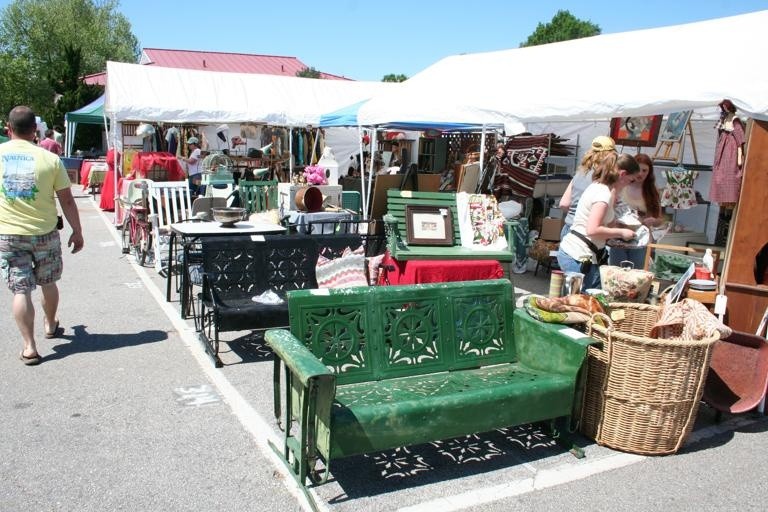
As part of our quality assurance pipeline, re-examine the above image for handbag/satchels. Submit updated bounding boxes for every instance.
[596,247,609,266]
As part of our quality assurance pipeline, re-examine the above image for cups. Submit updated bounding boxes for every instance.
[563,270,586,297]
[549,271,563,299]
[695,266,711,280]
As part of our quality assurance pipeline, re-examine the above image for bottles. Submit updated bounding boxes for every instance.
[702,249,713,276]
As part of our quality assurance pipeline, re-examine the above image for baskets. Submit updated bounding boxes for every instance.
[575,303,720,456]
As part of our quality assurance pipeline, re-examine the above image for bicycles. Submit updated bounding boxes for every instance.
[114,195,152,267]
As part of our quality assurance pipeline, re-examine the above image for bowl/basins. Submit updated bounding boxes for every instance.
[210,206,247,228]
[293,186,324,212]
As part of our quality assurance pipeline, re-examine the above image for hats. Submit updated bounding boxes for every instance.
[591,136,620,155]
[185,136,199,144]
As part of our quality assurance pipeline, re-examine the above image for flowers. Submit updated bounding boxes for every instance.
[303,165,329,185]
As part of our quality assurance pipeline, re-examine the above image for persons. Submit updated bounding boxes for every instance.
[557,133,621,247]
[175,136,206,197]
[709,99,744,211]
[554,152,639,295]
[609,155,663,270]
[0,106,88,366]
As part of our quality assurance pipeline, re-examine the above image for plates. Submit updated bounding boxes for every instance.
[687,280,717,291]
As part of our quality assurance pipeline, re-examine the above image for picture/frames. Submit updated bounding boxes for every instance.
[610,111,694,147]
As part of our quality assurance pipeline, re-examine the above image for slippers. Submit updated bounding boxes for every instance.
[42,315,59,338]
[19,348,38,366]
